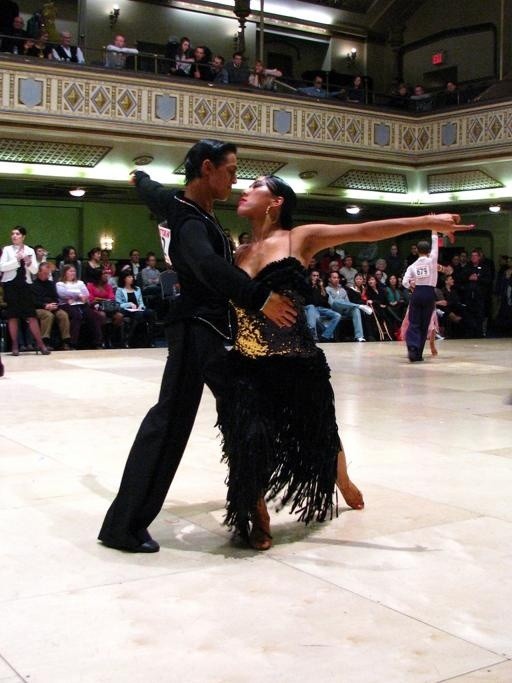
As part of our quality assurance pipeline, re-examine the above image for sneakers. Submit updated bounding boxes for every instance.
[359,304,373,315]
[356,337,366,341]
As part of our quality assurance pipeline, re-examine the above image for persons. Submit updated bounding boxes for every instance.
[169,37,281,90]
[95,137,299,557]
[0,226,51,356]
[230,173,477,552]
[224,228,251,253]
[300,76,362,102]
[446,81,468,108]
[105,34,138,67]
[435,246,512,341]
[307,243,420,342]
[0,12,85,64]
[30,245,180,351]
[392,84,432,112]
[401,212,440,363]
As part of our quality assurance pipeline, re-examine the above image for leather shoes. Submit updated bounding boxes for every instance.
[249,511,271,550]
[101,538,159,553]
[336,476,364,510]
[230,521,250,549]
[19,343,70,351]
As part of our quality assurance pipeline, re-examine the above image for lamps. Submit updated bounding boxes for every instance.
[109,4,120,29]
[344,48,357,63]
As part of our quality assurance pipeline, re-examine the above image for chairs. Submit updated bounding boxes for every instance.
[143,269,181,348]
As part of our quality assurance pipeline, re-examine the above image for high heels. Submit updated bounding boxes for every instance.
[35,343,50,355]
[12,350,18,355]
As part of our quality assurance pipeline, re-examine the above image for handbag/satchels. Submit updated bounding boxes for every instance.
[96,300,120,313]
